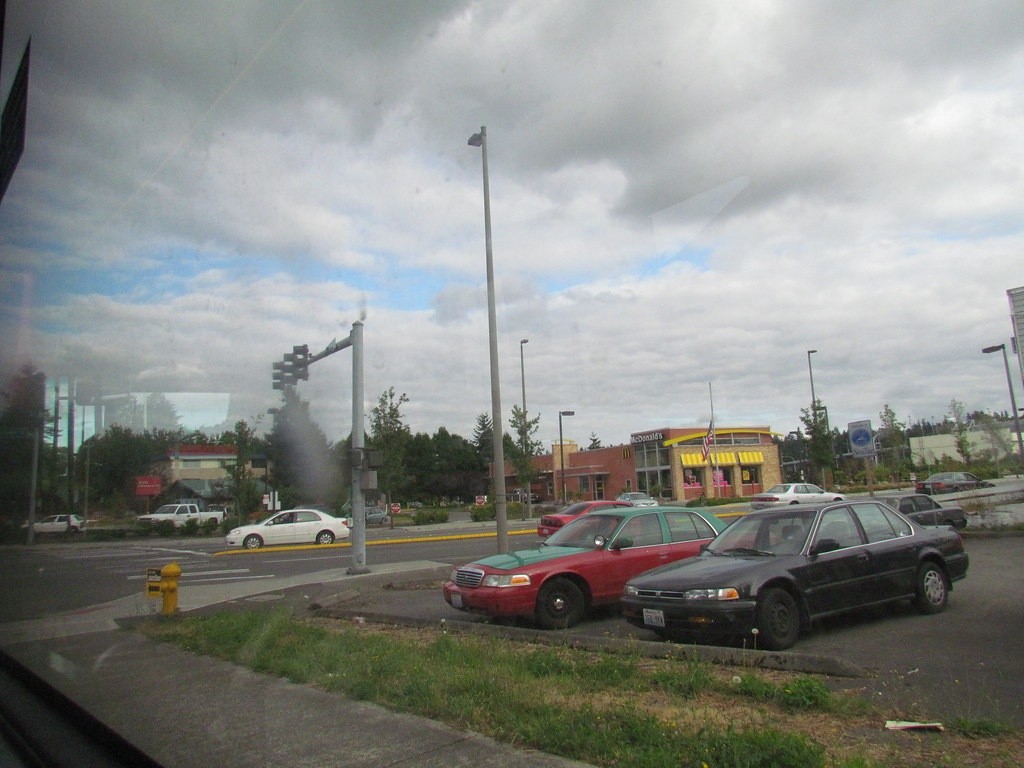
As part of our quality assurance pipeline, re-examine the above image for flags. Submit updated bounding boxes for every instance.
[701,421,713,460]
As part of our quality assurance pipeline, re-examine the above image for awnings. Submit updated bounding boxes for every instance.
[681,451,764,468]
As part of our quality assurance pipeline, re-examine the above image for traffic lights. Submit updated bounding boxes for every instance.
[292,345,309,381]
[272,361,285,391]
[283,353,298,386]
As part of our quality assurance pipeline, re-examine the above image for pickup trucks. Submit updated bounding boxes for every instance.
[136,503,222,536]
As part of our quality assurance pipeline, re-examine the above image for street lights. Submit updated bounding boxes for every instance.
[558,410,575,507]
[520,339,533,520]
[982,343,1024,470]
[808,349,826,492]
[467,125,510,555]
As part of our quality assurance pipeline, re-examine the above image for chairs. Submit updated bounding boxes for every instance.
[780,521,848,541]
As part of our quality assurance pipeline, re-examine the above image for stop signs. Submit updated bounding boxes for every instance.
[391,503,400,514]
[476,496,484,505]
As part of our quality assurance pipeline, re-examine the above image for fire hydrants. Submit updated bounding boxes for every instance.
[145,561,181,616]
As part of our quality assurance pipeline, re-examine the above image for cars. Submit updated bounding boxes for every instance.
[915,471,996,495]
[225,509,350,550]
[508,488,543,504]
[537,501,635,538]
[620,499,969,650]
[616,492,658,508]
[441,505,777,630]
[21,514,99,536]
[867,493,967,531]
[346,506,386,527]
[751,483,846,510]
